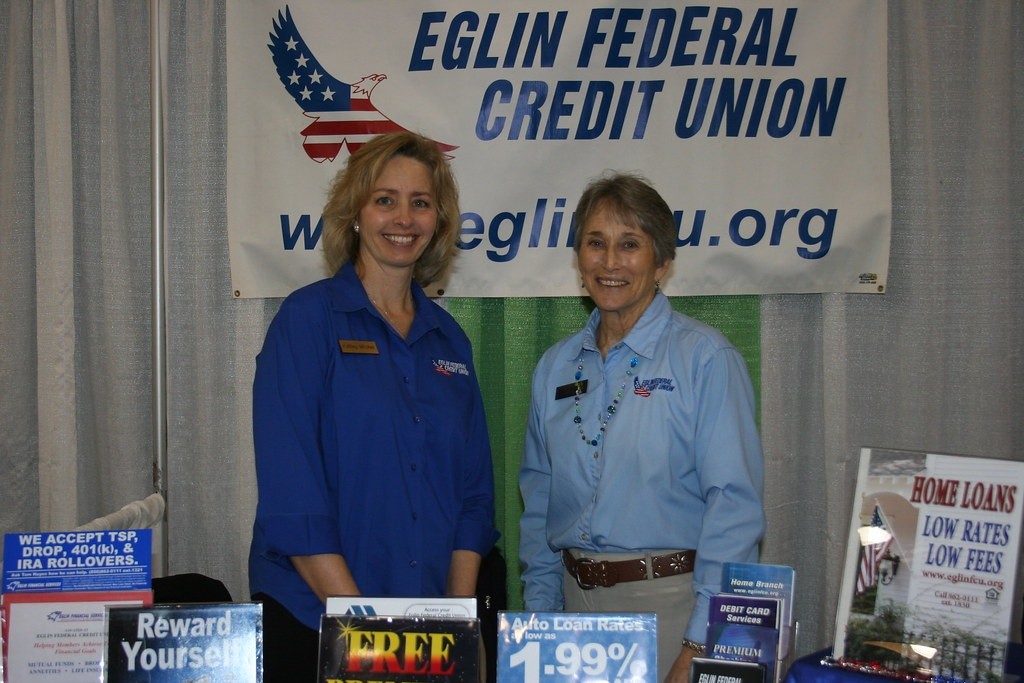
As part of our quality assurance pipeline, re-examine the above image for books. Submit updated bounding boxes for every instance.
[833,448,1024,683]
[690,562,796,683]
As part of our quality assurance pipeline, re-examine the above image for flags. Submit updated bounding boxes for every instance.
[855,504,910,596]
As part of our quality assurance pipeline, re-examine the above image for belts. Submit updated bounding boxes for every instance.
[561,546,697,590]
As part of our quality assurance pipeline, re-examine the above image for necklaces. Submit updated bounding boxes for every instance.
[572,347,642,458]
[367,291,410,340]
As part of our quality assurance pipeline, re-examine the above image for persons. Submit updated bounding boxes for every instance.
[248,133,509,683]
[515,174,763,681]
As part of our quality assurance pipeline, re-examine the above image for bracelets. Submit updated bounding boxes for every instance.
[682,640,706,652]
